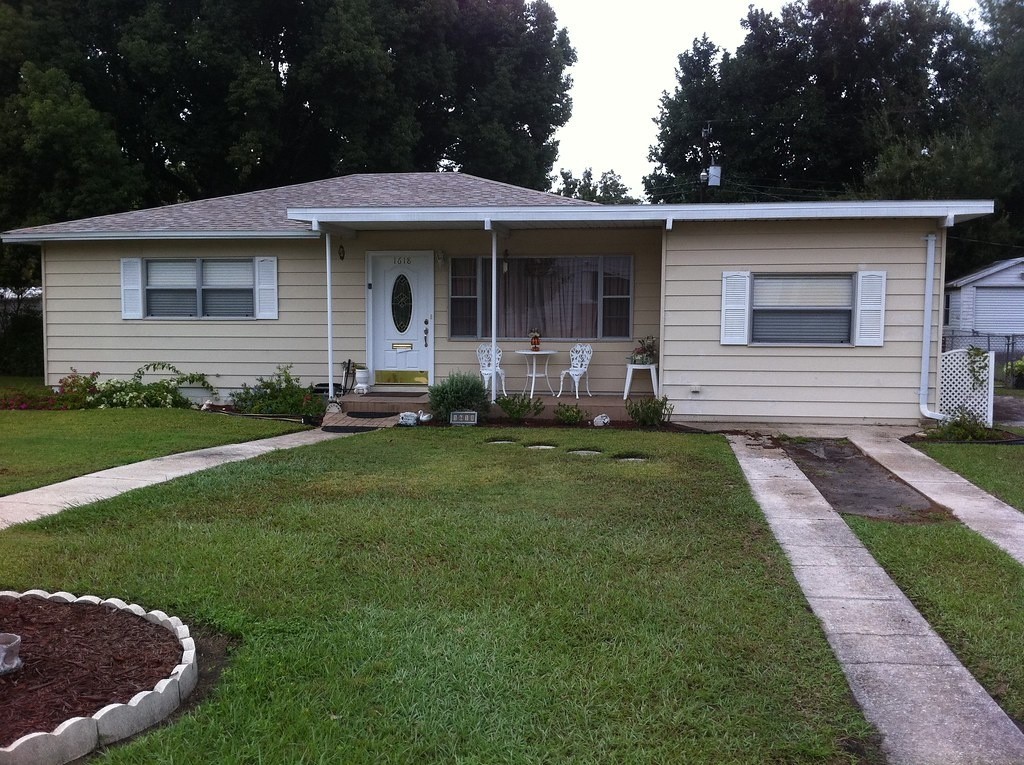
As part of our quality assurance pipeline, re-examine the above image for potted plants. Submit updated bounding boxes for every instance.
[353,365,371,395]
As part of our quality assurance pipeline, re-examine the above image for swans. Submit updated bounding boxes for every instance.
[418,410,434,422]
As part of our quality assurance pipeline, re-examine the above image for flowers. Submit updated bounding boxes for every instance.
[627,334,657,359]
[527,326,541,338]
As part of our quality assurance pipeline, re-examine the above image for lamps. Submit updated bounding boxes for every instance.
[338,245,345,261]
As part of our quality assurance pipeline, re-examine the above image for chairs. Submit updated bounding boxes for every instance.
[557,343,594,399]
[477,343,509,397]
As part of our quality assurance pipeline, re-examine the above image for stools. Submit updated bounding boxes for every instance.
[623,363,659,401]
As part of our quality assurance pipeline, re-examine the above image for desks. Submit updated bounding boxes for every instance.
[514,350,559,400]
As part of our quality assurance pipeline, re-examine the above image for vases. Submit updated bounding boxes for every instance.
[530,336,541,351]
[643,352,655,364]
